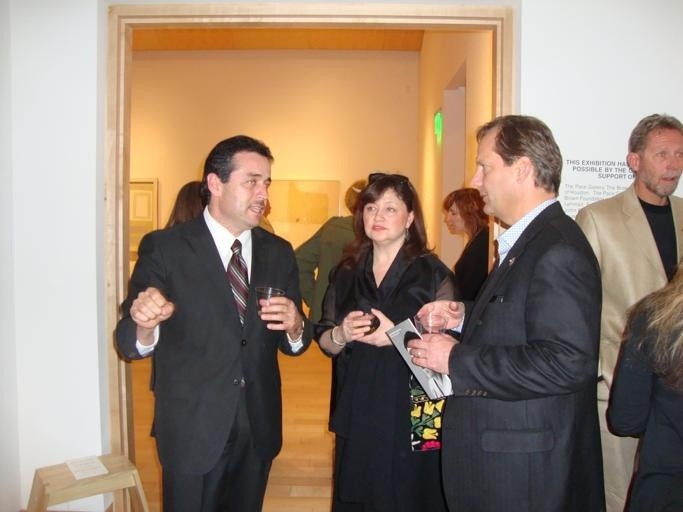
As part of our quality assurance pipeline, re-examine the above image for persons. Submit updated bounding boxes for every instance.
[402,329,453,398]
[572,113,682,512]
[310,170,463,512]
[440,187,491,305]
[403,111,608,511]
[291,178,382,433]
[113,134,317,512]
[145,179,212,440]
[602,259,683,512]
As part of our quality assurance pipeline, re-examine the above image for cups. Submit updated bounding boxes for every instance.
[412,312,449,336]
[253,287,286,315]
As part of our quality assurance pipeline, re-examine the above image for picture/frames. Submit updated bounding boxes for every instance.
[129,178,158,280]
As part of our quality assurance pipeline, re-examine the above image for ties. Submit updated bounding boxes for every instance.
[486,240,500,275]
[226,240,249,323]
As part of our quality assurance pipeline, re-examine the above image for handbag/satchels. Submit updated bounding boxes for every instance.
[407,373,445,453]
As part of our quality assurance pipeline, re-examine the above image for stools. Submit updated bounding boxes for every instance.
[27,454,149,512]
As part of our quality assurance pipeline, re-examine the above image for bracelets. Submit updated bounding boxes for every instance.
[285,321,306,344]
[330,324,346,348]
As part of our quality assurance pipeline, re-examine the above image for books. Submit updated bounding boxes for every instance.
[384,318,455,401]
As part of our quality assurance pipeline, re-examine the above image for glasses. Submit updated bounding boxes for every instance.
[368,172,409,186]
